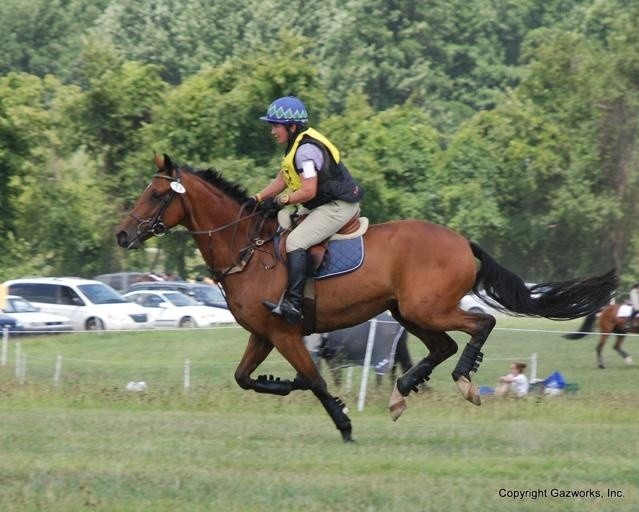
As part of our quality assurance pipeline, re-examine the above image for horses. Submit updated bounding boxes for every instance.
[115,148,621,443]
[563,303,639,369]
[312,315,413,386]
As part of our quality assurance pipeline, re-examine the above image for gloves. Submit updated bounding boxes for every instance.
[241,195,258,216]
[262,197,279,212]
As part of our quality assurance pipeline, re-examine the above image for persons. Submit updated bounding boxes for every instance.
[625,284,639,328]
[495,362,529,399]
[251,96,364,318]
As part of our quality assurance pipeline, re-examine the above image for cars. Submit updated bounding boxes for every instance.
[457,280,547,317]
[0,294,76,334]
[92,268,238,327]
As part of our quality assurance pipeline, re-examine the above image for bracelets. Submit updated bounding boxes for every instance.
[255,194,261,203]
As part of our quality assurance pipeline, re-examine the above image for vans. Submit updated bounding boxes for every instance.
[3,274,155,331]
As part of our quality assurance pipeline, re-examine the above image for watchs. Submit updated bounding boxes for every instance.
[279,194,289,205]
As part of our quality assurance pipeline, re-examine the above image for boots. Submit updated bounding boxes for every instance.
[261,248,308,325]
[625,309,638,329]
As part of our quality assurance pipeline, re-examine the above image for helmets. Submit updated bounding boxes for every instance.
[259,95,309,128]
